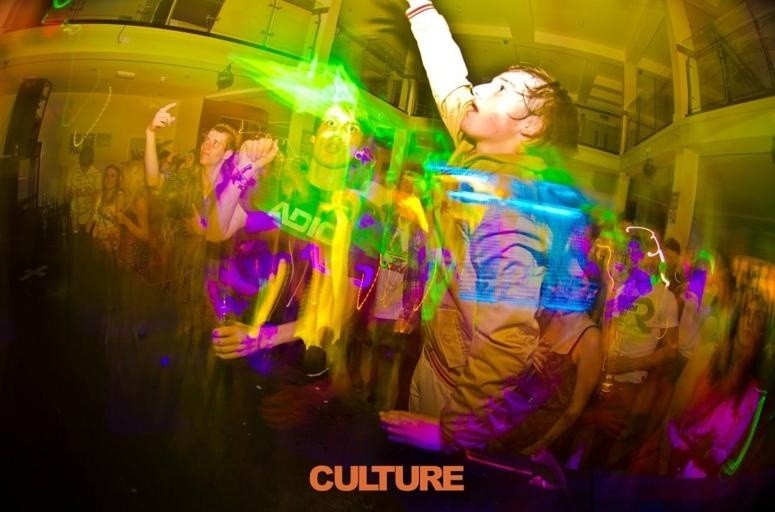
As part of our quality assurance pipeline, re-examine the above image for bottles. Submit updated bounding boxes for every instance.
[211,287,237,328]
[598,365,618,398]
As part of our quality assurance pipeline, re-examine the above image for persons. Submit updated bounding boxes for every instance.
[62,0,775,512]
